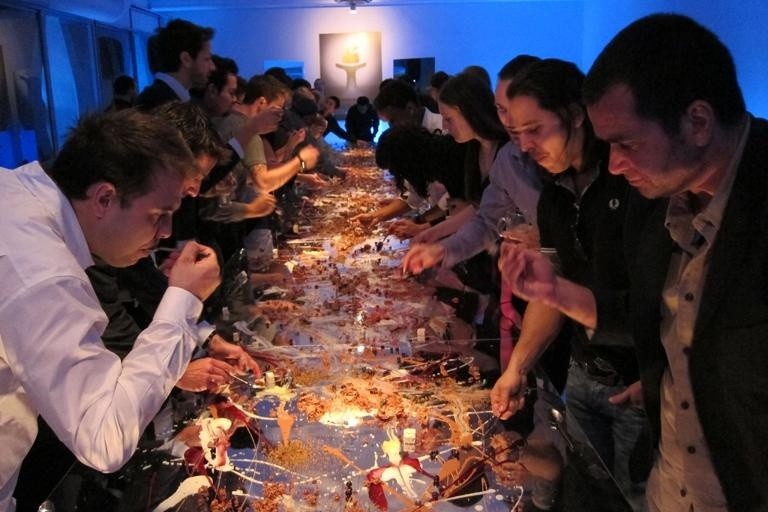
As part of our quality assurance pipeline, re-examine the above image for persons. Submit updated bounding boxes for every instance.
[371,12,767,511]
[500,315,514,374]
[2,18,367,509]
[345,96,379,142]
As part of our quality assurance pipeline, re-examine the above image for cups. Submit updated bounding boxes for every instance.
[496,208,541,244]
[244,227,273,270]
[215,183,234,215]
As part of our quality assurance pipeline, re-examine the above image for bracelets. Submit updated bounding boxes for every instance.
[500,301,511,305]
[500,326,511,331]
[202,331,216,349]
[297,155,306,172]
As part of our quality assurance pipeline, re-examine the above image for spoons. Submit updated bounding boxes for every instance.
[374,230,393,253]
[545,406,577,454]
[145,245,206,254]
[228,373,266,391]
